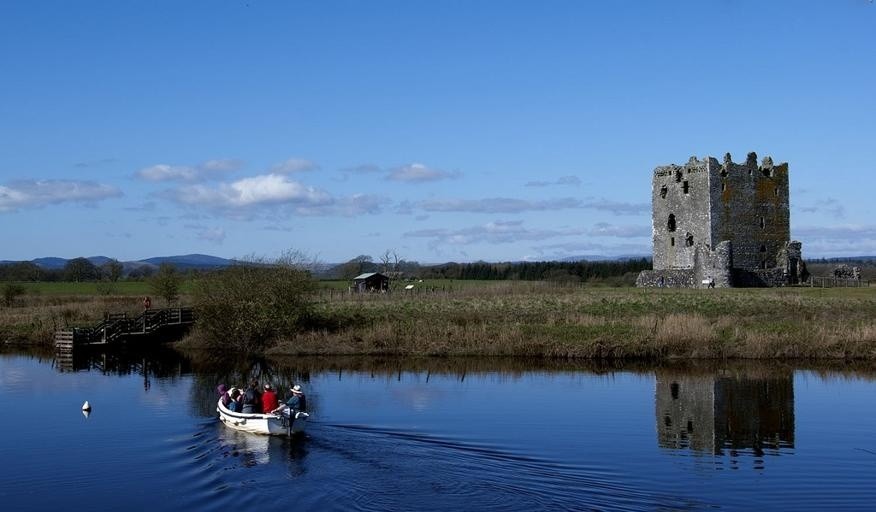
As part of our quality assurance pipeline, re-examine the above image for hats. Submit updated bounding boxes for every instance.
[289,385,303,394]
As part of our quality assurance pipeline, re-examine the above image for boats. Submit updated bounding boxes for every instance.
[217,421,282,468]
[215,384,308,439]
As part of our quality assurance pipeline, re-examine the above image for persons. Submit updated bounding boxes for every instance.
[143,297,151,310]
[707,276,716,288]
[218,380,305,414]
